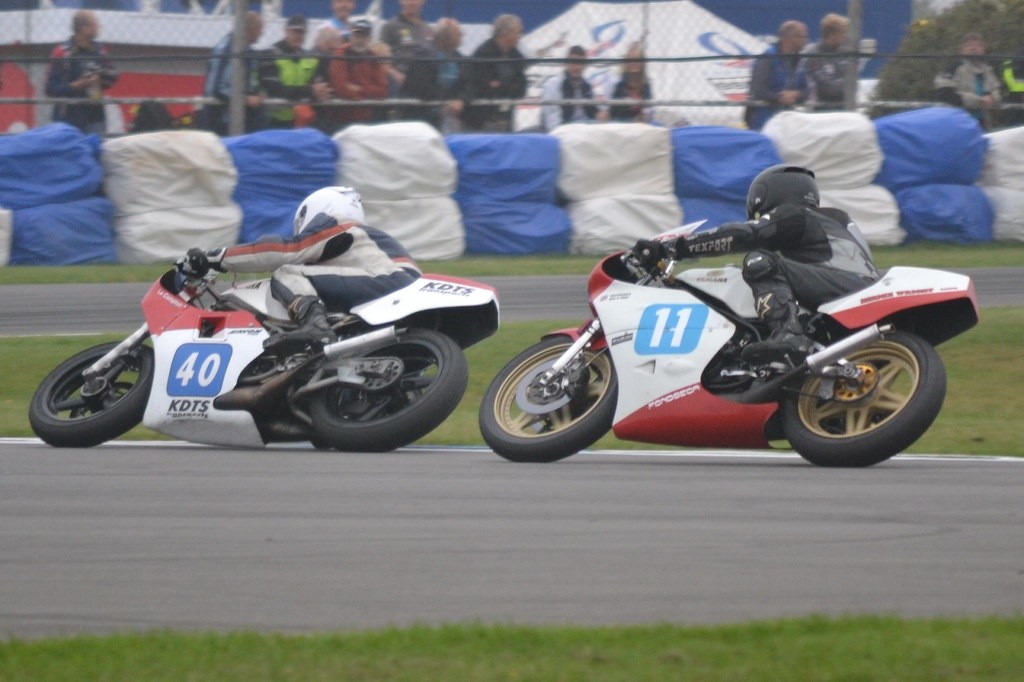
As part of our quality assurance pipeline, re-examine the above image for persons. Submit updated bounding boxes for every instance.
[932,32,1024,133]
[202,0,652,134]
[631,165,879,366]
[178,186,422,354]
[45,10,120,133]
[745,13,851,131]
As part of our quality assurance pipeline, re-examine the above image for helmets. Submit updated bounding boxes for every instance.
[746,164,821,221]
[293,186,364,237]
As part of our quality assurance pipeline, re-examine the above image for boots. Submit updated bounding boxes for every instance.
[263,295,339,351]
[741,291,814,364]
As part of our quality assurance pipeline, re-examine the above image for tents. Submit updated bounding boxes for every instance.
[515,0,773,125]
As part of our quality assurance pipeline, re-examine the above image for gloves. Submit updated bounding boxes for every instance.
[173,247,228,277]
[633,235,685,266]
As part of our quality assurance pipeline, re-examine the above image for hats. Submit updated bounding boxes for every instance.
[350,19,372,30]
[284,15,307,33]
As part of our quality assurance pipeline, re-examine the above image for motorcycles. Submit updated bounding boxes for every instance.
[27,247,501,452]
[478,220,979,467]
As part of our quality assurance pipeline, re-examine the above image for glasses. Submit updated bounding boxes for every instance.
[795,33,809,39]
